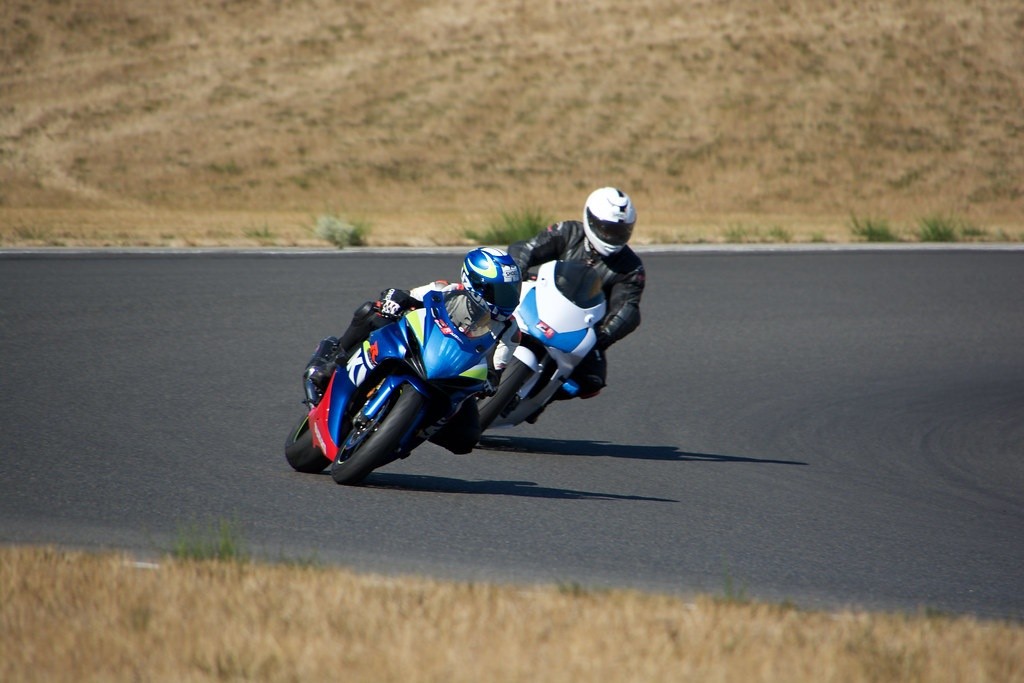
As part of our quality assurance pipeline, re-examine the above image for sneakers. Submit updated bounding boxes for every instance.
[310,338,348,389]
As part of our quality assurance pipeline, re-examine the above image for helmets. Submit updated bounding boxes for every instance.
[459,246,523,322]
[581,187,638,256]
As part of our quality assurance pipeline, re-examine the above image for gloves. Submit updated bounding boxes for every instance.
[365,286,411,332]
[593,332,616,353]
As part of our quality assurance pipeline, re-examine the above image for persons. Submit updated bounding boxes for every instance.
[310,247,521,455]
[508,186,646,424]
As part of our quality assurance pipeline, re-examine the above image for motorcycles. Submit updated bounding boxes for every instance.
[473,255,609,441]
[285,286,498,486]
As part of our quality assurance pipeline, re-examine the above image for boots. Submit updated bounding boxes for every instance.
[473,366,506,400]
[524,384,574,424]
